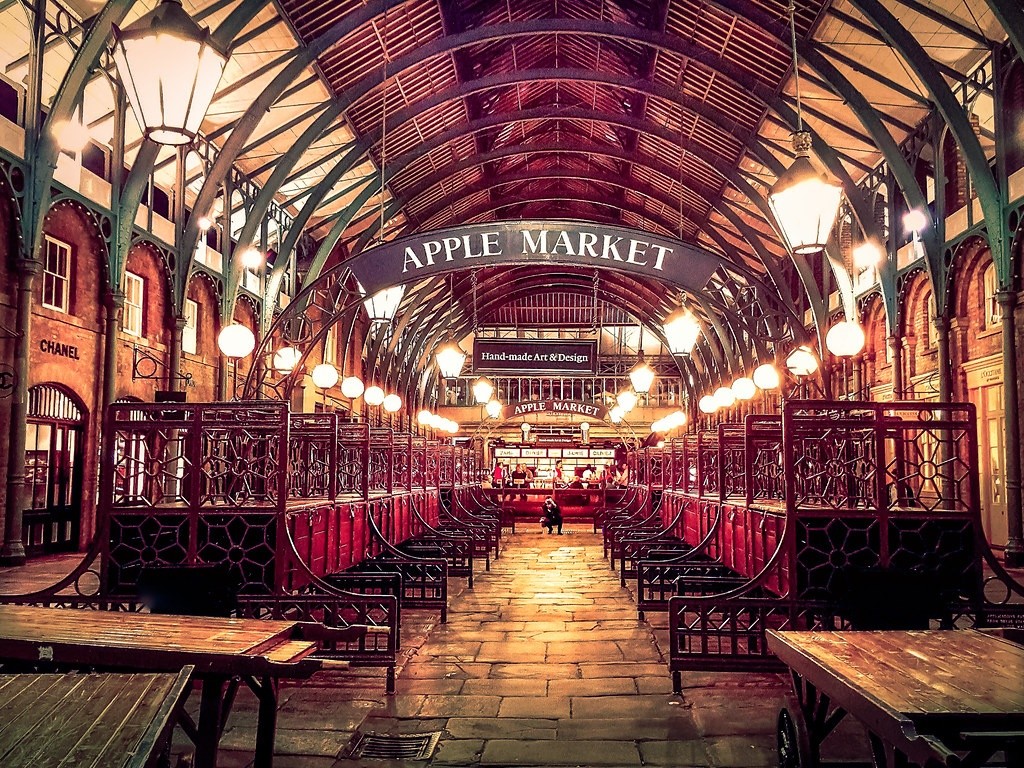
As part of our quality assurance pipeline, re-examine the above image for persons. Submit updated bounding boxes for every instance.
[600,463,629,489]
[491,462,504,487]
[512,463,533,488]
[554,459,563,487]
[542,496,563,535]
[582,464,593,479]
[887,468,917,507]
[570,476,584,489]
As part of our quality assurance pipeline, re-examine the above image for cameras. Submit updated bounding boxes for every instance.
[547,501,551,505]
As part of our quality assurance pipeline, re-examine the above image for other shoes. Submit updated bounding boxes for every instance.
[548,528,553,534]
[557,532,563,535]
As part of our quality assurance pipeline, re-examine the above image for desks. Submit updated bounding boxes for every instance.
[763,625,1024,768]
[0,663,196,768]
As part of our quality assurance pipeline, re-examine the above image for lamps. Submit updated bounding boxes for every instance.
[485,395,504,418]
[272,349,401,428]
[364,1,406,323]
[659,62,702,357]
[699,364,779,425]
[218,319,256,399]
[608,197,657,425]
[432,141,468,379]
[106,0,230,147]
[767,1,843,258]
[788,315,871,403]
